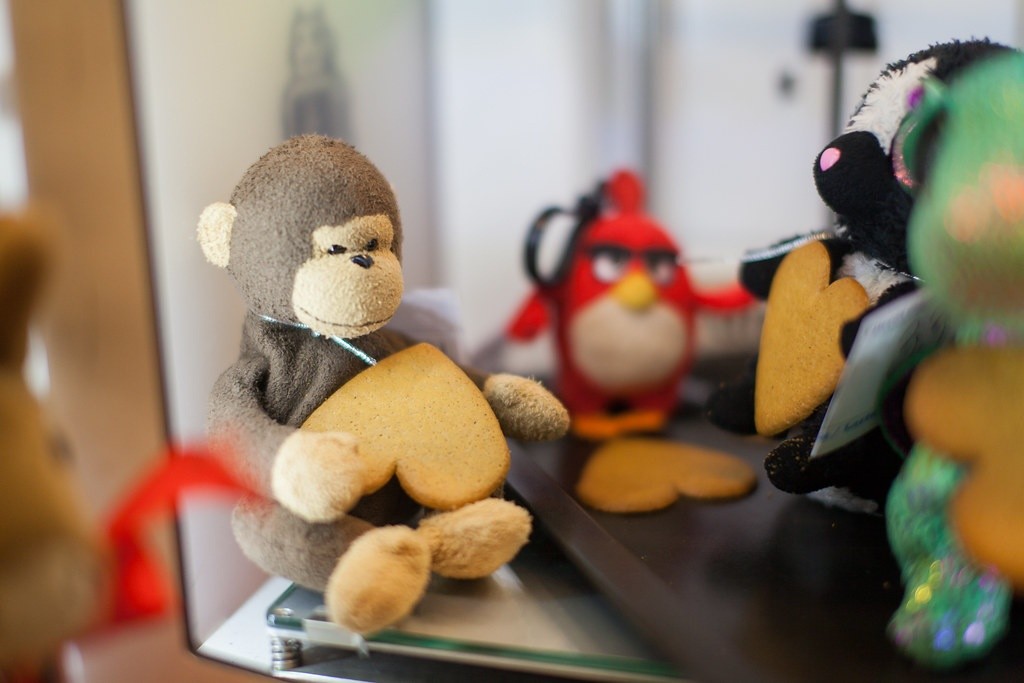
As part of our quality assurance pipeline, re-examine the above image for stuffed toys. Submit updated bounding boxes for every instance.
[199,131,571,635]
[700,39,1021,495]
